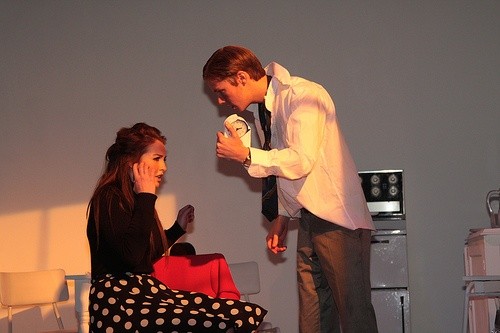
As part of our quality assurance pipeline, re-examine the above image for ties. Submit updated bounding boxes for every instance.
[258,75,278,222]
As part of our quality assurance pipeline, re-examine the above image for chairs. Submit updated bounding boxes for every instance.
[0,268,69,332]
[228,261,260,304]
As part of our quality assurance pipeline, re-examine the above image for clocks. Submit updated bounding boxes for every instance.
[223,113,252,151]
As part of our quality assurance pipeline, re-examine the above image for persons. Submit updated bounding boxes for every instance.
[85,123,268,333]
[202,46,380,333]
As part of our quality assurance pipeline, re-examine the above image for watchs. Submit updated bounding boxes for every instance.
[243,152,251,167]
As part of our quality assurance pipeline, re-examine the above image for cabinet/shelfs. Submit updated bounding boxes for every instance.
[368,218,410,333]
[465,229,500,333]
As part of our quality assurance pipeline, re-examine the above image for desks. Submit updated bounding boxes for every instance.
[152,253,241,302]
